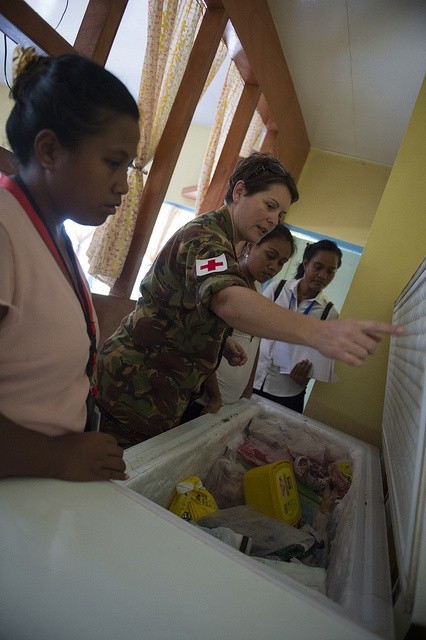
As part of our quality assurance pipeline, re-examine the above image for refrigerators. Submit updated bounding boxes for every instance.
[2,257,425,639]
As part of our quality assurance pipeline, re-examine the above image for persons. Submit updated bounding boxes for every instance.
[177,224,299,426]
[253,239,343,414]
[84,149,405,451]
[1,43,141,482]
